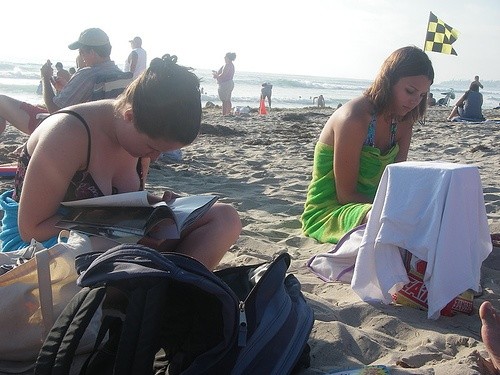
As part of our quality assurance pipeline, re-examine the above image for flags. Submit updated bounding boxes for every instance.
[425,12,461,56]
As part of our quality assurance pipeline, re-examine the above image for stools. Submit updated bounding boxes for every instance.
[350,161,492,319]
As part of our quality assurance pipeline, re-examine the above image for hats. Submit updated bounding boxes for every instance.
[68,28,110,50]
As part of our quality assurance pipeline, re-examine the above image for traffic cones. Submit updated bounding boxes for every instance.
[259,99,267,114]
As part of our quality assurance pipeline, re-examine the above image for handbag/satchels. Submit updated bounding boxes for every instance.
[0,231,81,363]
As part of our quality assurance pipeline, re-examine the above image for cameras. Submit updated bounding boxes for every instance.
[51,75,59,84]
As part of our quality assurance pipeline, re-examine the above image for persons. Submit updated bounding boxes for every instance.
[479,301,500,373]
[0,28,486,139]
[0,55,242,271]
[299,46,435,243]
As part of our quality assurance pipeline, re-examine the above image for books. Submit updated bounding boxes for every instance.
[54,191,219,243]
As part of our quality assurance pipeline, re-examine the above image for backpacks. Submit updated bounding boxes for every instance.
[33,243,315,375]
